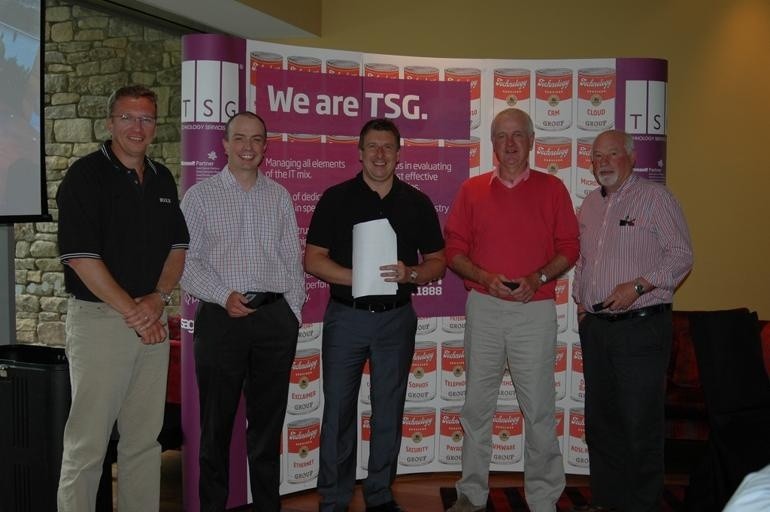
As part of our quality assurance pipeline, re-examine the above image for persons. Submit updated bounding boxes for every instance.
[303,118,447,512]
[576,130,696,512]
[443,108,580,512]
[55,83,190,512]
[179,110,308,512]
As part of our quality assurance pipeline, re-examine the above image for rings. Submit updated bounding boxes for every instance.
[144,314,150,322]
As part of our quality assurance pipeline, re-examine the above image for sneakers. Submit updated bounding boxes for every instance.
[446,498,487,511]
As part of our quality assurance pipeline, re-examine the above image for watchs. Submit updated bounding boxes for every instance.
[408,266,419,284]
[536,269,549,287]
[632,279,647,297]
[152,289,174,308]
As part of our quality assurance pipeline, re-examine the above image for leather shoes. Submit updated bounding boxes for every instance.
[366,501,407,511]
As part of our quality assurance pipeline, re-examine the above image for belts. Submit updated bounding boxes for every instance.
[598,303,671,321]
[330,293,410,311]
[259,292,282,305]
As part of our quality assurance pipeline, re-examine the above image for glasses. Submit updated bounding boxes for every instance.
[109,113,155,125]
